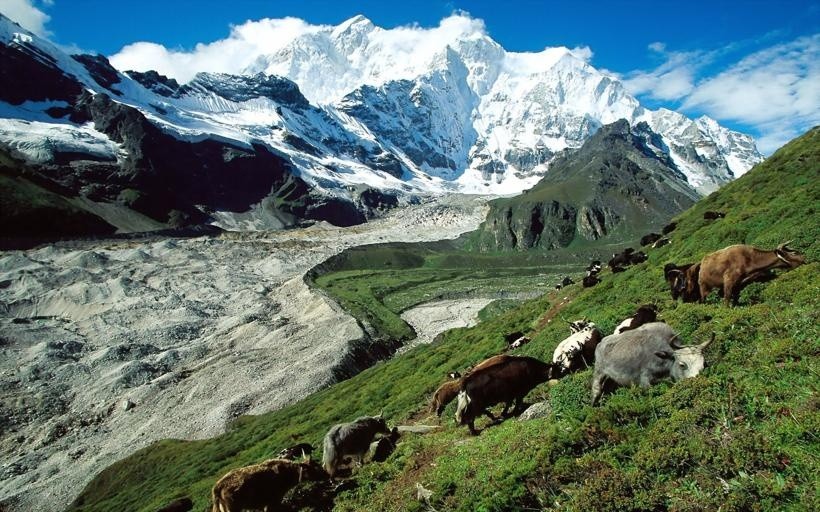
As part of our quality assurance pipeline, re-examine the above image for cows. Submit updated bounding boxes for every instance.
[277,442,318,461]
[431,302,717,436]
[211,457,331,512]
[321,409,391,488]
[155,497,194,512]
[553,211,809,308]
